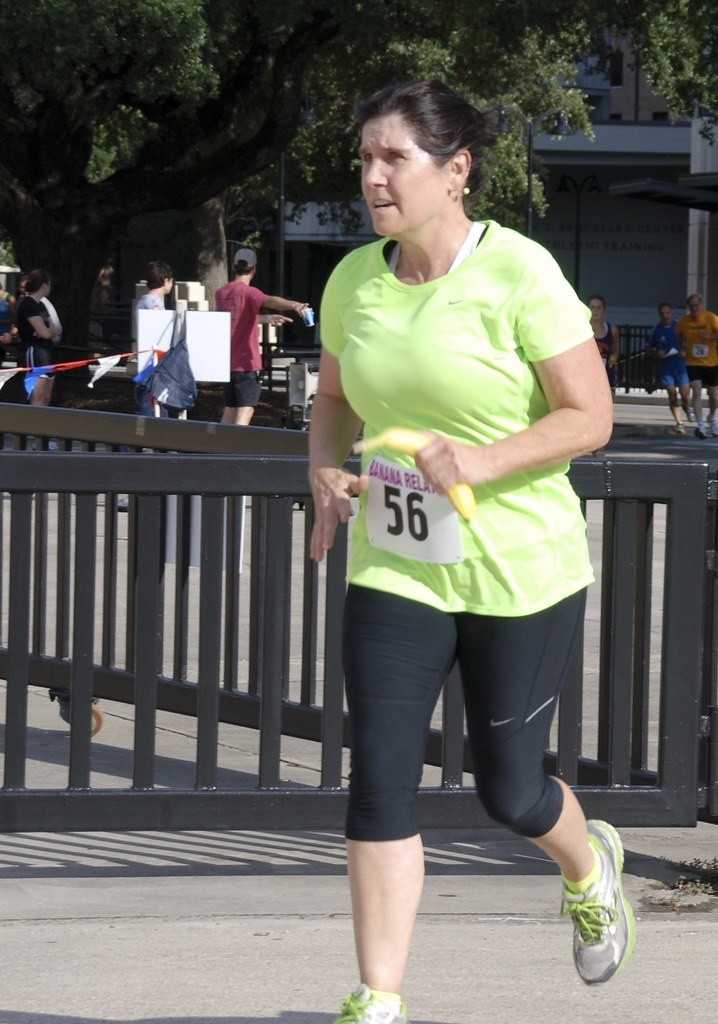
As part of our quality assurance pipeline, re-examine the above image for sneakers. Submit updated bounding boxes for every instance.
[561,819,636,986]
[707,415,718,437]
[682,406,696,422]
[695,426,707,440]
[333,984,409,1024]
[676,424,685,433]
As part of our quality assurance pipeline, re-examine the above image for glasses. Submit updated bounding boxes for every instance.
[48,282,53,286]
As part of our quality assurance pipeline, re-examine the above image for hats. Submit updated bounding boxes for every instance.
[235,249,257,267]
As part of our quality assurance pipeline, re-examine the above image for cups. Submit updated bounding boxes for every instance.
[303,308,314,327]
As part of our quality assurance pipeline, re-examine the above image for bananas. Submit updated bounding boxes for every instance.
[351,428,475,520]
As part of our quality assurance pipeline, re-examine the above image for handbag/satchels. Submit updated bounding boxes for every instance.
[146,311,196,409]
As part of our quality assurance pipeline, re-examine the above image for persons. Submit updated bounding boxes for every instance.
[588,293,718,439]
[0,248,311,425]
[308,80,633,1024]
